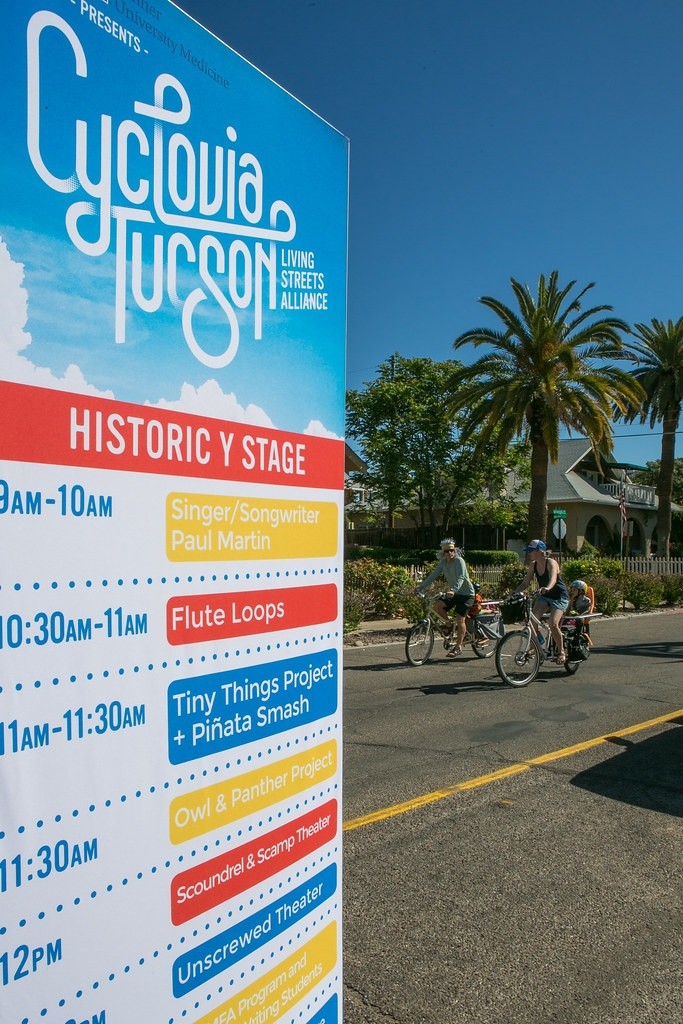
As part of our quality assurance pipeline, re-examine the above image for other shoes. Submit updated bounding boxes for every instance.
[440,626,453,637]
[446,643,462,657]
[526,650,536,659]
[556,652,567,664]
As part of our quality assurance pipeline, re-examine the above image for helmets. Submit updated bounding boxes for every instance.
[471,581,482,590]
[571,580,587,594]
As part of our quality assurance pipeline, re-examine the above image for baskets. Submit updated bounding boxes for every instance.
[499,594,527,625]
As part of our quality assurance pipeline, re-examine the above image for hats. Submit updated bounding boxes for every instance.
[523,540,546,552]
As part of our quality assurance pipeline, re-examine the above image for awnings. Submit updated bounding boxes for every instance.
[603,462,654,486]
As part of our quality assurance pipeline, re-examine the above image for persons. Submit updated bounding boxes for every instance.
[566,580,591,642]
[412,539,473,658]
[467,583,491,618]
[509,539,568,665]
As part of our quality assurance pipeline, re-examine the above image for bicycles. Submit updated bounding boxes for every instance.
[405,591,505,668]
[493,587,604,688]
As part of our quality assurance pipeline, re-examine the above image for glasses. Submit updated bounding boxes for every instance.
[443,549,455,554]
